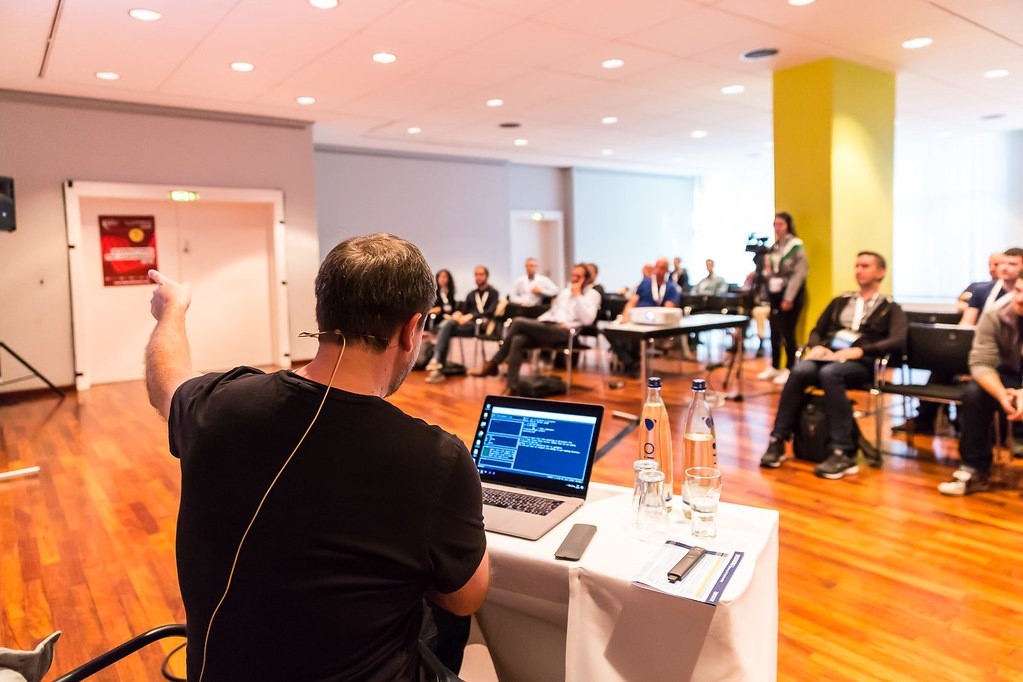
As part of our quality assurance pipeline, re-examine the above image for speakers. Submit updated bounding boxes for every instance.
[0,176,17,233]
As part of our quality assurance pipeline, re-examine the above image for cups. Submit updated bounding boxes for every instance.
[685,466,721,535]
[630,460,671,537]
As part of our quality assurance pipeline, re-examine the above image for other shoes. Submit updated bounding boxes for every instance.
[468,362,499,377]
[503,384,519,396]
[426,362,443,371]
[937,469,988,496]
[425,372,445,383]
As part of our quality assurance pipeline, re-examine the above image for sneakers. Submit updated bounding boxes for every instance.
[760,439,786,467]
[814,449,859,480]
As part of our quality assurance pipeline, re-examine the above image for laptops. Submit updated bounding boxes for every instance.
[470,394,605,541]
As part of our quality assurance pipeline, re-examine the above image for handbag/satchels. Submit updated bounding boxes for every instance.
[518,375,567,397]
[792,392,859,463]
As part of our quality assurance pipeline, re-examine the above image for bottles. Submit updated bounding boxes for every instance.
[638,377,674,512]
[684,379,717,519]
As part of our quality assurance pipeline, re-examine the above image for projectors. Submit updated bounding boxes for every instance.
[630,307,683,325]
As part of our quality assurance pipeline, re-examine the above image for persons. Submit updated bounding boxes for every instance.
[468,264,601,398]
[642,264,654,280]
[424,266,498,384]
[892,248,1023,432]
[537,264,606,371]
[938,269,1023,496]
[422,270,456,334]
[613,259,679,378]
[957,251,1002,315]
[760,251,907,480]
[144,232,490,682]
[690,259,728,295]
[503,258,559,323]
[670,259,688,292]
[764,212,806,384]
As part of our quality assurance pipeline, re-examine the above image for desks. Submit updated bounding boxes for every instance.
[473,480,781,682]
[599,314,751,410]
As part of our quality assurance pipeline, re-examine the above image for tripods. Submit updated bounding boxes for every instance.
[722,253,768,390]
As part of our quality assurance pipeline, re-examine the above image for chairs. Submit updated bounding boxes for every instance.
[45,624,466,682]
[422,285,1023,488]
